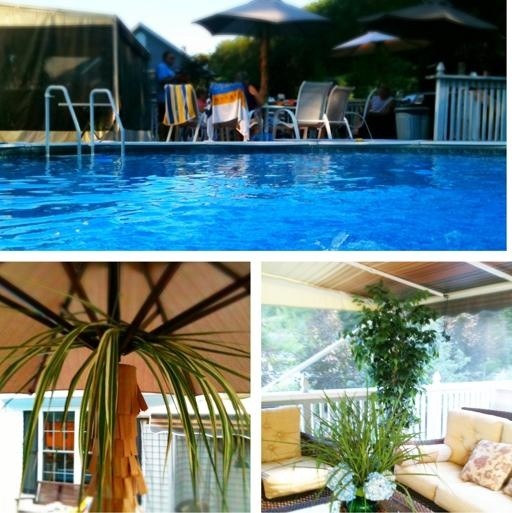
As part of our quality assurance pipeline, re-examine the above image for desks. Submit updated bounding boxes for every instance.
[261,105,297,142]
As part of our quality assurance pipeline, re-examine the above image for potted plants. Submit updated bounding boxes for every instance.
[261,368,440,512]
[340,278,439,472]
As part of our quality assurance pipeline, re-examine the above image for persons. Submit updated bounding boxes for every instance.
[154,48,191,141]
[229,68,267,140]
[350,82,397,139]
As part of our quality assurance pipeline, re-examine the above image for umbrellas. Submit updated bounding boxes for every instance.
[328,27,430,89]
[190,0,335,94]
[354,0,499,73]
[1,260,250,398]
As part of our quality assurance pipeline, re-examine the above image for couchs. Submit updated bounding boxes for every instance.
[393,408,512,512]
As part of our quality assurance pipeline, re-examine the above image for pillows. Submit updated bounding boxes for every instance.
[503,477,512,497]
[460,439,512,492]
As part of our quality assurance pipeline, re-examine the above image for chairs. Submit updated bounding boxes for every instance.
[16,481,81,511]
[303,85,355,140]
[162,83,207,141]
[261,404,340,503]
[271,80,334,141]
[345,88,379,140]
[193,82,263,142]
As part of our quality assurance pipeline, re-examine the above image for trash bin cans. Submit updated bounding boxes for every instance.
[394,104,431,139]
